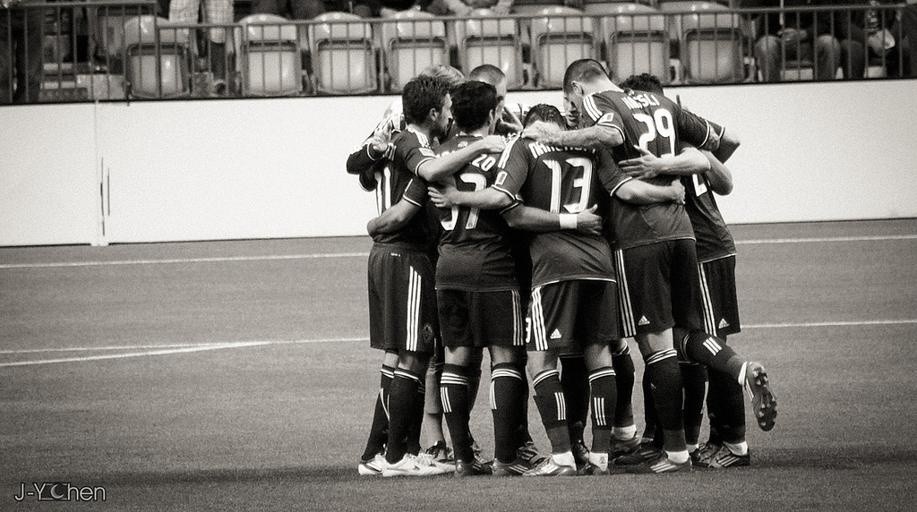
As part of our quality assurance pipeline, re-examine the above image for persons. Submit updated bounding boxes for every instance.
[1,0,917,105]
[339,105,778,481]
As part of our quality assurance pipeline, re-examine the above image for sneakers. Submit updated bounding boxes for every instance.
[744,360,777,430]
